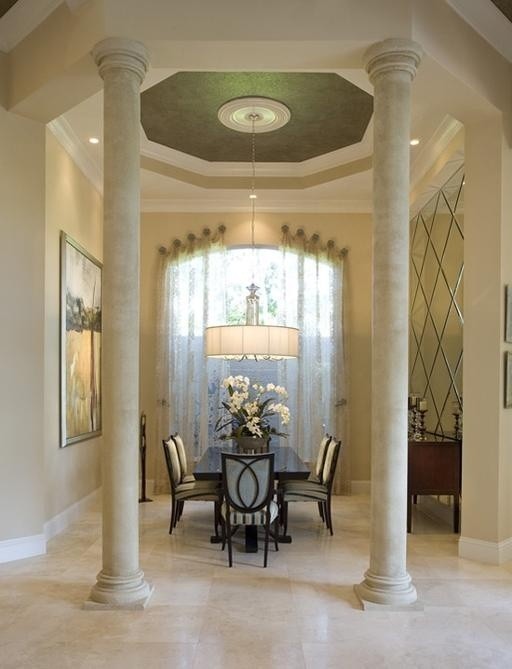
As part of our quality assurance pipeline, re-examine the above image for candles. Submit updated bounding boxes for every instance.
[420,401,426,411]
[411,397,416,405]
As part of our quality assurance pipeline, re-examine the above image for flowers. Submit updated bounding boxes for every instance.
[214,374,291,441]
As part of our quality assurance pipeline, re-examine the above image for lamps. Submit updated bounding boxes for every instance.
[204,94,301,363]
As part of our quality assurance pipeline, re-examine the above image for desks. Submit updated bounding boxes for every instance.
[408,428,462,534]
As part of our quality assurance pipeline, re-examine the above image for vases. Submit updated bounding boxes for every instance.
[238,436,272,455]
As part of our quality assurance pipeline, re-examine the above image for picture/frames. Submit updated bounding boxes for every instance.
[59,230,104,448]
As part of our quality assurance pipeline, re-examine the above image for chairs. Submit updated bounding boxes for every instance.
[162,431,224,536]
[170,432,225,521]
[279,436,342,536]
[214,451,279,568]
[278,433,332,526]
